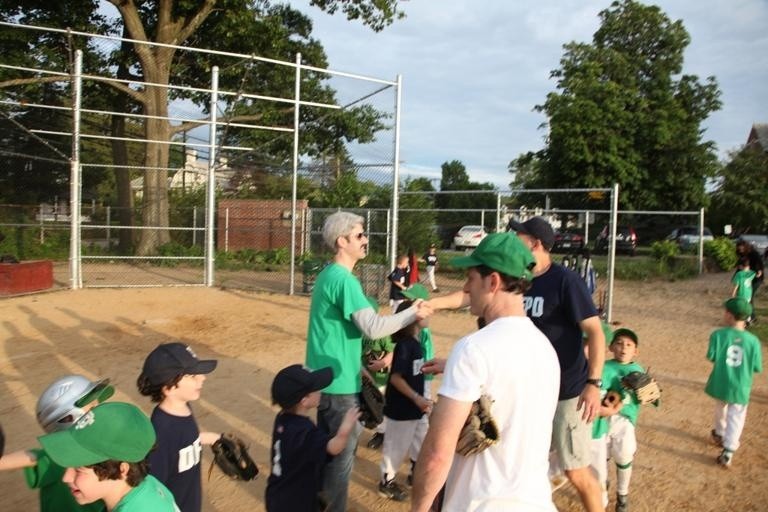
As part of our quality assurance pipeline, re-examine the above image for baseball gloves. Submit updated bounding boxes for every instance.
[622,370,662,406]
[365,349,389,375]
[457,396,500,457]
[213,432,260,482]
[357,377,385,430]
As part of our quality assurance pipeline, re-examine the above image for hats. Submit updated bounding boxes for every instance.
[449,217,554,282]
[399,284,428,301]
[37,402,157,468]
[144,343,217,386]
[725,298,753,319]
[76,385,113,406]
[271,364,333,401]
[609,328,638,345]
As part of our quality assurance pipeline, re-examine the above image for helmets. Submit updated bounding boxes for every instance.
[36,377,108,431]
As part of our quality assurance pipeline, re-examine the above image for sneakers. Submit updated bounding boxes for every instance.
[379,459,415,500]
[712,429,733,467]
[368,432,383,448]
[616,495,627,512]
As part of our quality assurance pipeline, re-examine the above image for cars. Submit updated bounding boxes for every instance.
[666,224,714,250]
[728,225,768,257]
[595,223,637,256]
[551,228,585,253]
[452,224,487,247]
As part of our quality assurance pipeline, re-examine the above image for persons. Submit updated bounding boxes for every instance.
[35,400,181,512]
[726,237,765,324]
[306,210,436,512]
[264,364,365,512]
[360,243,440,451]
[378,299,437,502]
[1,374,116,512]
[416,217,607,512]
[134,340,258,512]
[551,319,662,511]
[703,298,764,471]
[408,230,562,511]
